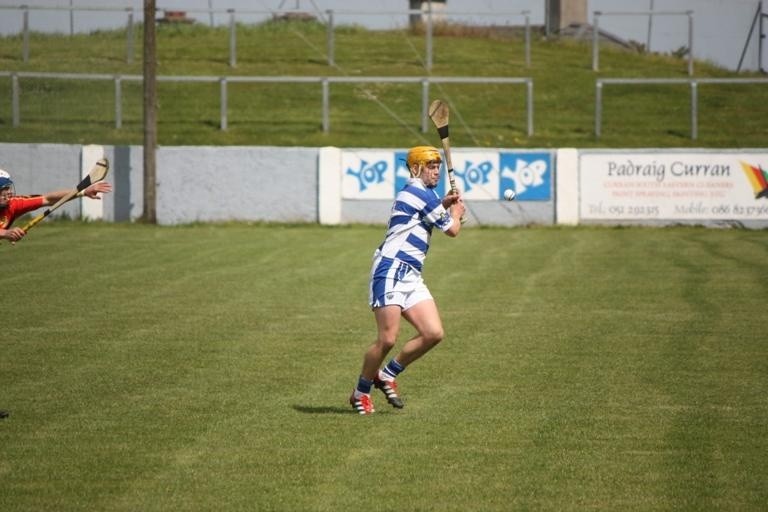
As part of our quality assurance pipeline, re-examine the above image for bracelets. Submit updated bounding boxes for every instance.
[76,188,85,198]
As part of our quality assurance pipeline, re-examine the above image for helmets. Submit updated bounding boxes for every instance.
[0,169,15,210]
[406,146,442,178]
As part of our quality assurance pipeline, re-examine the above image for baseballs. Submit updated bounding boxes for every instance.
[504,190,516,201]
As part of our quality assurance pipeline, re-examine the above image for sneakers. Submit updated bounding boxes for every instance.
[375,370,404,408]
[350,388,376,415]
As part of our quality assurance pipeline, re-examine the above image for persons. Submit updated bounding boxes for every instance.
[349,144,466,416]
[0,156,113,246]
